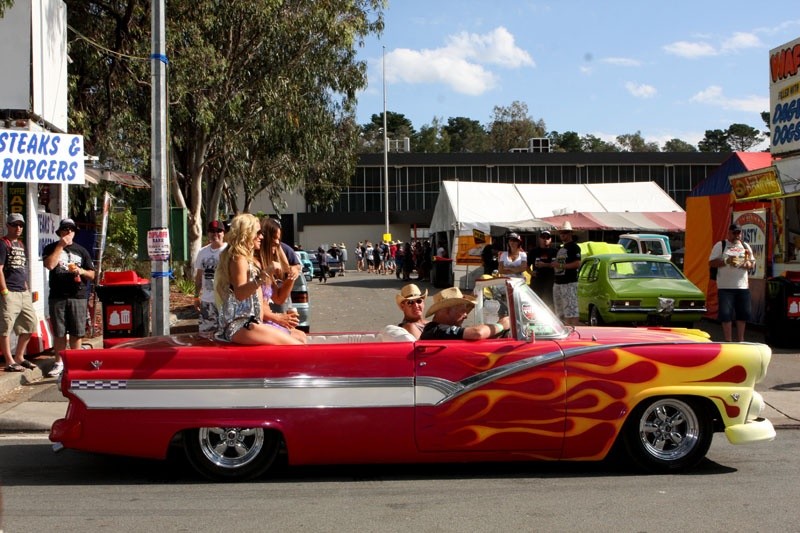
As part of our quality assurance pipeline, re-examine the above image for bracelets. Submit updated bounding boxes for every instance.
[492,322,506,334]
[252,277,260,288]
[724,257,728,265]
[0,288,9,295]
[193,292,199,297]
[80,267,85,275]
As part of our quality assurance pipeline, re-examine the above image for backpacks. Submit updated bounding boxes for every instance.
[709,240,749,280]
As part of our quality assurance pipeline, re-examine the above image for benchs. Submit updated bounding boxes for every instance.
[380,324,417,342]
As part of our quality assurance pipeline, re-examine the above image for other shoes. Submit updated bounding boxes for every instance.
[319,267,429,285]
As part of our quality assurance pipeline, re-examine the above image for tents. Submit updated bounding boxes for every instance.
[425,151,782,290]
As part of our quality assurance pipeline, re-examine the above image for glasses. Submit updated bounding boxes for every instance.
[541,235,550,239]
[509,238,518,242]
[8,222,24,228]
[401,299,422,305]
[559,231,569,234]
[257,230,262,235]
[210,229,222,233]
[730,231,741,234]
[63,227,73,231]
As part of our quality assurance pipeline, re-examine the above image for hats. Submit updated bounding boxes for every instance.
[425,287,478,318]
[539,230,552,237]
[549,221,577,234]
[207,221,225,232]
[729,224,742,231]
[55,219,76,236]
[7,213,25,224]
[331,240,404,248]
[509,233,522,241]
[396,283,428,307]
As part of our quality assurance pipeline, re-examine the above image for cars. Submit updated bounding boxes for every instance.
[560,242,707,327]
[289,250,342,332]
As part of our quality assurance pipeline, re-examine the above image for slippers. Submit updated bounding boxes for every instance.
[20,359,36,368]
[4,363,25,372]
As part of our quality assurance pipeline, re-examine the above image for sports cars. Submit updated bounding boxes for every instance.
[49,274,778,475]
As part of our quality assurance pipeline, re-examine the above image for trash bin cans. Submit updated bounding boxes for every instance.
[432,256,453,289]
[95,270,151,347]
[764,271,800,350]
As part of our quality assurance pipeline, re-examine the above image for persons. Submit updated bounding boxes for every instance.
[479,217,581,324]
[193,218,230,331]
[251,218,300,312]
[394,283,428,342]
[211,213,311,346]
[417,284,513,342]
[40,215,96,380]
[269,218,303,312]
[291,233,447,282]
[708,223,758,345]
[1,213,38,374]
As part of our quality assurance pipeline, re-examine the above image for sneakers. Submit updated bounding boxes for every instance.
[47,362,63,376]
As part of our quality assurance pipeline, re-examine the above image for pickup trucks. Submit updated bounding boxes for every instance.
[618,234,684,276]
